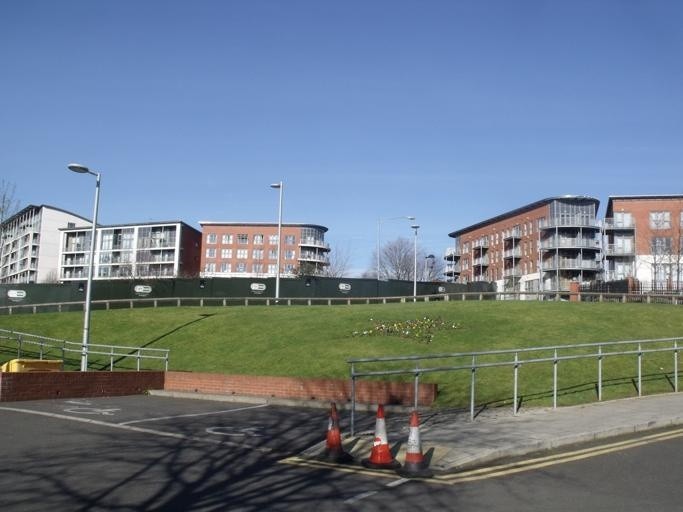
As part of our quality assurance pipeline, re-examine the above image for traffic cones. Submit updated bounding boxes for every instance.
[316,401,355,464]
[361,404,402,471]
[398,411,435,480]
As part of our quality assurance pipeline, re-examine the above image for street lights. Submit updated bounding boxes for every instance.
[375,213,416,281]
[65,161,105,373]
[269,180,285,304]
[411,224,420,302]
